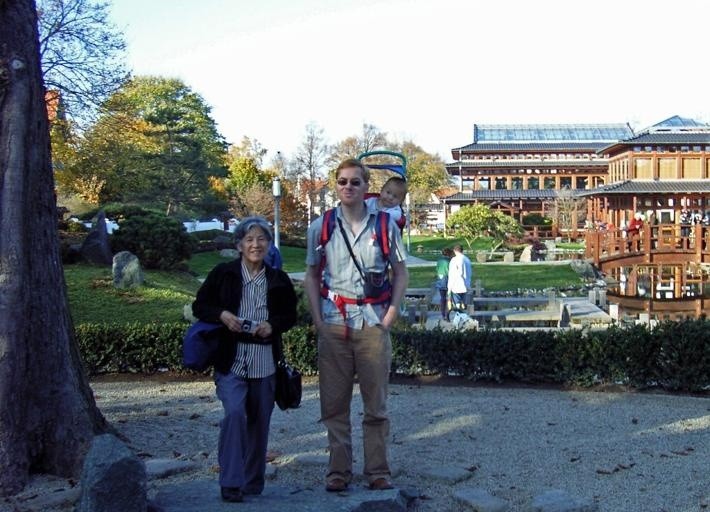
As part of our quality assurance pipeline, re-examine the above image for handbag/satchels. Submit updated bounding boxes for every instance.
[364,271,390,298]
[276,358,304,411]
[181,319,223,372]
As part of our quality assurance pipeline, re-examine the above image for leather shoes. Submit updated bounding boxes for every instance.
[325,478,347,492]
[367,478,394,490]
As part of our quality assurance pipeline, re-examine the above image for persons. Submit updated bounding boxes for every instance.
[627,212,646,253]
[650,212,659,236]
[691,213,702,226]
[679,212,690,236]
[679,205,691,221]
[596,220,606,231]
[447,244,472,310]
[363,174,407,236]
[302,158,411,495]
[701,211,710,226]
[191,216,299,504]
[433,247,453,321]
[261,239,283,270]
[583,219,595,229]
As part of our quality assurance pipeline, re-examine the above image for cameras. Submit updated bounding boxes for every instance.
[235,317,258,334]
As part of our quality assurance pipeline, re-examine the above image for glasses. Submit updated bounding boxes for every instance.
[336,177,362,186]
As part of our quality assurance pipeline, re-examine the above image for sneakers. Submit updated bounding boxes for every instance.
[220,484,263,504]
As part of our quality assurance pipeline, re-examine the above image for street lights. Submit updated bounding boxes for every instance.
[405,193,411,252]
[272,177,282,254]
